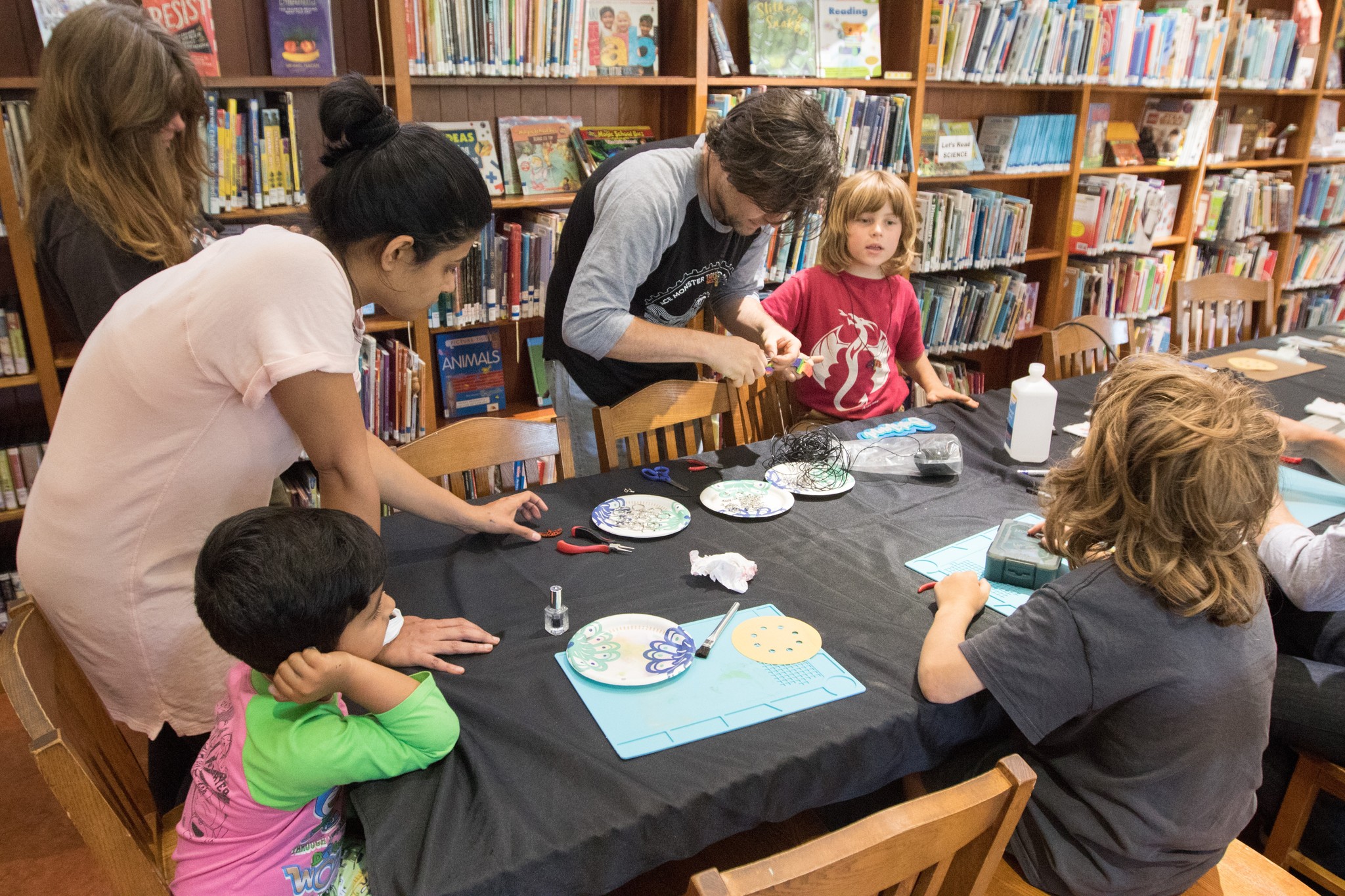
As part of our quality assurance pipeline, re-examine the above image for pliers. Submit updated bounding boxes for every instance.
[687,459,724,471]
[917,580,938,593]
[557,525,636,554]
[712,358,772,383]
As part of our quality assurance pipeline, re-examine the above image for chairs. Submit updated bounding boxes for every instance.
[736,369,794,445]
[593,378,745,474]
[390,416,574,503]
[686,749,1345,896]
[1170,273,1274,353]
[0,595,184,896]
[1042,315,1135,380]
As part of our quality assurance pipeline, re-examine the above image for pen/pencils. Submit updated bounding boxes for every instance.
[1017,469,1052,476]
[1026,487,1053,498]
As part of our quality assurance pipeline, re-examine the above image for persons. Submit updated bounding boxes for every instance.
[760,169,980,436]
[1236,406,1345,850]
[28,0,221,345]
[15,71,548,820]
[540,87,844,479]
[914,352,1275,896]
[169,506,461,896]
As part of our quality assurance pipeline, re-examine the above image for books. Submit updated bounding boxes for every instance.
[402,0,660,78]
[707,1,740,77]
[926,0,1345,93]
[141,0,221,79]
[747,0,816,76]
[811,0,882,80]
[31,1,110,50]
[265,0,338,78]
[1,88,307,220]
[287,89,1344,521]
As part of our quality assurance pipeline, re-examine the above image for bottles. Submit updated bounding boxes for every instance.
[1004,362,1058,463]
[543,585,570,636]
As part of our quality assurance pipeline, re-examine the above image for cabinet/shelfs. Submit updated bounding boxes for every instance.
[0,0,1345,518]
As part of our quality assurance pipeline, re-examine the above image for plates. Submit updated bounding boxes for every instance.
[700,480,794,518]
[764,461,856,496]
[566,613,696,687]
[591,494,691,538]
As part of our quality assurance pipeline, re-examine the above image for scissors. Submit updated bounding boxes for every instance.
[642,466,689,492]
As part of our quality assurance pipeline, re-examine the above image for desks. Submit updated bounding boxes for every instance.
[341,323,1345,896]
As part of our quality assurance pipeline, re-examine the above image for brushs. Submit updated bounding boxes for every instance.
[696,600,740,657]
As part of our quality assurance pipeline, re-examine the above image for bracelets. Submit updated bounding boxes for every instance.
[382,607,405,647]
